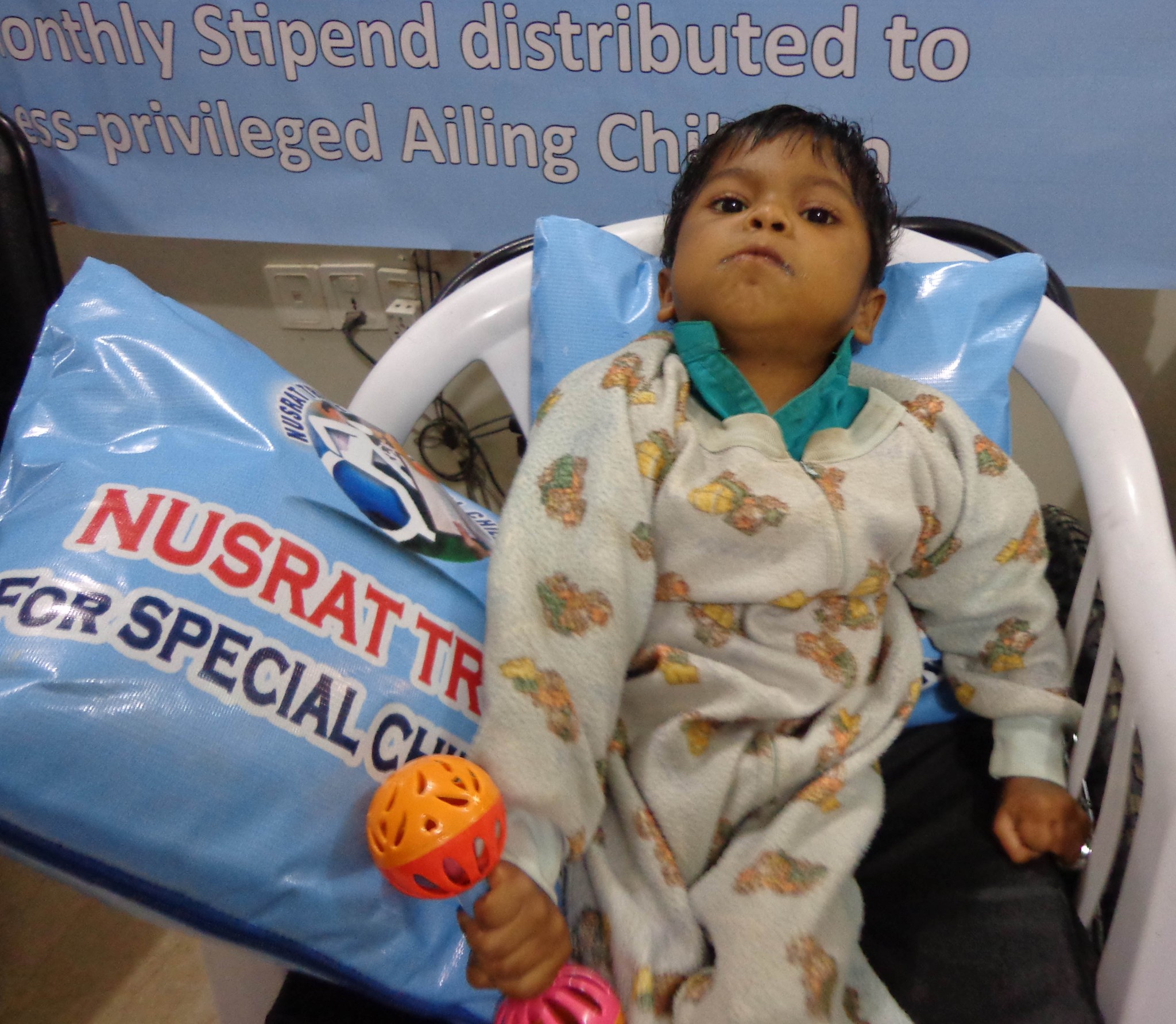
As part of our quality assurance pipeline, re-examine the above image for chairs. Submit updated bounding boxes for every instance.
[192,210,1176,1024]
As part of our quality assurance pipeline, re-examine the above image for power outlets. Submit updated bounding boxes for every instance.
[318,265,438,332]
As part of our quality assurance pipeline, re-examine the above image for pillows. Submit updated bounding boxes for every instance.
[527,215,1049,729]
[0,258,566,1024]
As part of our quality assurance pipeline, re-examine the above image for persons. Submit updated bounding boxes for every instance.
[457,102,1089,1024]
